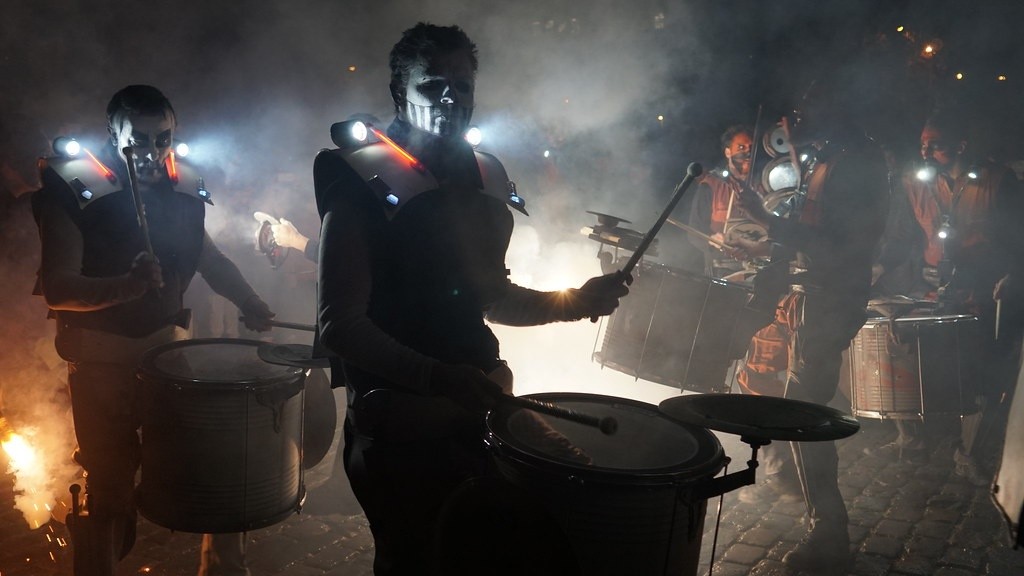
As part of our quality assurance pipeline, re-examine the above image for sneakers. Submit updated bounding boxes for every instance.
[782,518,855,568]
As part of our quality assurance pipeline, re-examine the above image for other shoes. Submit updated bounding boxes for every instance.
[863,441,920,463]
[955,462,989,486]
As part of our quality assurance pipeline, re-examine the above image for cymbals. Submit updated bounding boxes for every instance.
[304,369,336,470]
[657,392,863,442]
[585,209,633,226]
[868,293,940,321]
[257,342,331,368]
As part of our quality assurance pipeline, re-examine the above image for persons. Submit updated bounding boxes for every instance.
[0,83,391,576]
[313,21,634,576]
[462,88,1024,487]
[736,71,893,568]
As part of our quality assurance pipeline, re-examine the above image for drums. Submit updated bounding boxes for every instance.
[599,255,752,394]
[481,390,730,575]
[848,313,982,420]
[724,120,802,248]
[132,336,307,532]
